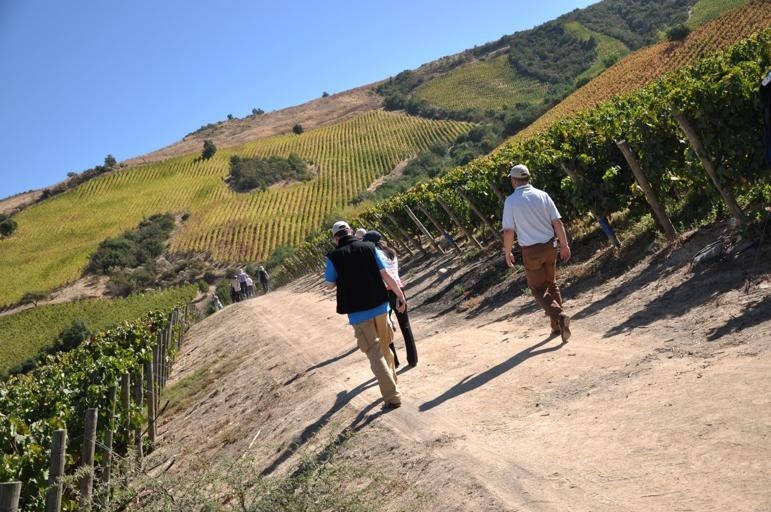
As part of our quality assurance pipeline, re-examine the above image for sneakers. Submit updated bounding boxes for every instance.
[551,327,560,334]
[382,403,401,409]
[559,312,571,343]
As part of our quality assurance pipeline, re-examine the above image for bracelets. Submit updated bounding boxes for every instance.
[559,243,569,248]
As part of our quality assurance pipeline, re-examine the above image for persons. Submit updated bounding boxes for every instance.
[503,164,573,342]
[212,292,224,311]
[363,231,419,369]
[229,265,270,304]
[353,228,397,332]
[325,221,408,409]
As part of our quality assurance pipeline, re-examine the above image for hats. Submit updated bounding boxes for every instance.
[507,163,531,182]
[356,228,367,239]
[363,230,382,241]
[333,220,350,236]
